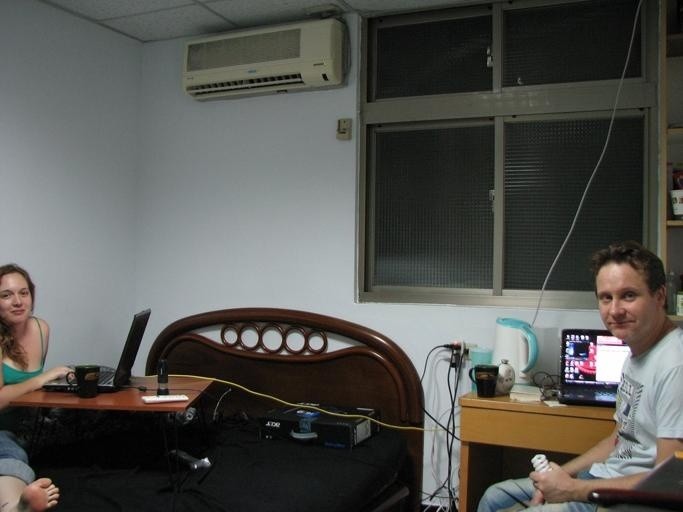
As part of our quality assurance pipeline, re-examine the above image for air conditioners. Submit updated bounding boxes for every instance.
[182,18,348,102]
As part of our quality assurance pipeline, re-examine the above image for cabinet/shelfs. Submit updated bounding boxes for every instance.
[658,1,682,323]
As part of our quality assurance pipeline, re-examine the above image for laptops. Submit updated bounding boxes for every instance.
[559,329,631,408]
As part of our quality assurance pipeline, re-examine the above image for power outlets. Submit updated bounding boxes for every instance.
[464,343,477,360]
[336,118,352,140]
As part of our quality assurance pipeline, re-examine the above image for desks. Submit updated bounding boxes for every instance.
[456,390,616,512]
[9,375,215,492]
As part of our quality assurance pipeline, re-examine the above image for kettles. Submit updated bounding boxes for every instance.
[491,316,539,387]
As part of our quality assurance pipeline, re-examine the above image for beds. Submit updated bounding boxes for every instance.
[29,308,425,512]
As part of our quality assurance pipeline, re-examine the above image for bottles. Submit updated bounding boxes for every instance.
[496,359,516,396]
[156,359,169,396]
[667,161,683,187]
[667,271,678,316]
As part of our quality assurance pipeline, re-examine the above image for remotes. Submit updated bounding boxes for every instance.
[142,395,189,404]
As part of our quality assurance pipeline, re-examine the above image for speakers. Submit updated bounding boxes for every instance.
[42,310,150,392]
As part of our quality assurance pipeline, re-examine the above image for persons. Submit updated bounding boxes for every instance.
[479,236,682,512]
[0,264,64,512]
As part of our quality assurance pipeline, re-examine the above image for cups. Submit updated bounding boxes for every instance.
[669,187,683,220]
[469,348,493,393]
[468,363,498,400]
[65,364,100,399]
